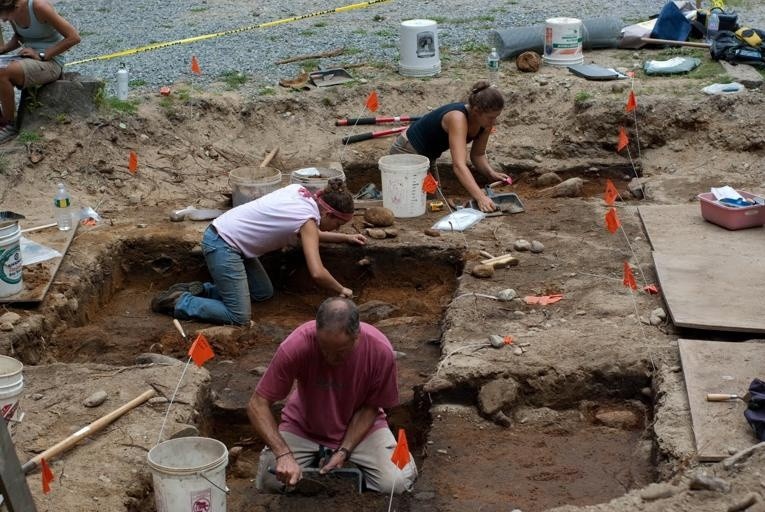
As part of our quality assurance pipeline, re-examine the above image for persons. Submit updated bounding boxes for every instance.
[245,295,420,498]
[0,0,81,146]
[150,177,368,326]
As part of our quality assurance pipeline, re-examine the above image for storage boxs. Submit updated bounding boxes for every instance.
[697,188,764,232]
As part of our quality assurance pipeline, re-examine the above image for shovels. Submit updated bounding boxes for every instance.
[466,184,526,217]
[487,173,521,187]
[267,466,327,492]
[282,449,363,496]
[708,390,752,405]
[310,64,355,88]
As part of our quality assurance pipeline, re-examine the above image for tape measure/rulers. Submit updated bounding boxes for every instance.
[429,201,443,212]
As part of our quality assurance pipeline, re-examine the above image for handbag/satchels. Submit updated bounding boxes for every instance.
[697,7,738,40]
[650,1,693,49]
[709,26,765,65]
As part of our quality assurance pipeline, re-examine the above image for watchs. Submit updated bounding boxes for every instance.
[39,51,46,62]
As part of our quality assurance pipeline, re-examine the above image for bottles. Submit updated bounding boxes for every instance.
[486,45,501,86]
[115,59,130,100]
[706,9,720,44]
[54,182,72,230]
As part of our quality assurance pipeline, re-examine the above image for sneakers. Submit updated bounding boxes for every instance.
[0,118,17,145]
[150,291,184,317]
[355,192,378,202]
[352,181,382,201]
[168,281,209,298]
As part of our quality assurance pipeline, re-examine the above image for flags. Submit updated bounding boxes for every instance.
[189,57,202,73]
[186,334,213,367]
[418,170,440,196]
[390,82,510,213]
[615,128,630,151]
[389,428,411,468]
[363,91,386,112]
[620,259,639,291]
[39,459,54,494]
[624,91,640,115]
[127,152,138,172]
[603,207,624,235]
[603,180,617,206]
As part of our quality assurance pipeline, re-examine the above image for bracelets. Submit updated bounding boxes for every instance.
[275,451,293,460]
[330,446,351,462]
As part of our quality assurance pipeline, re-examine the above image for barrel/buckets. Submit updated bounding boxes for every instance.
[0,210,24,300]
[0,355,25,447]
[541,17,585,68]
[227,165,283,208]
[397,18,443,78]
[377,152,432,221]
[288,166,348,196]
[146,435,229,512]
